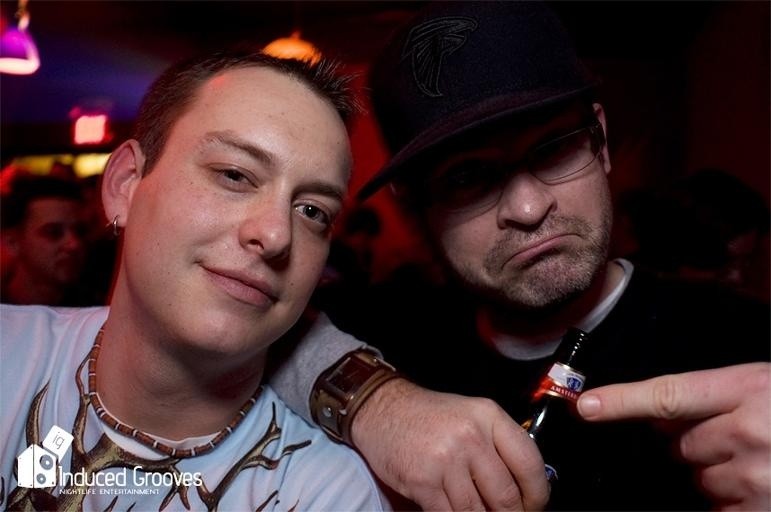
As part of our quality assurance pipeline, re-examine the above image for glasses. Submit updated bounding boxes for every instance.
[407,124,603,211]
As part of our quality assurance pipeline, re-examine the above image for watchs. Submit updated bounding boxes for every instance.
[308,350,401,446]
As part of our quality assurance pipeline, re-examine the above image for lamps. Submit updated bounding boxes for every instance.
[0,0,40,75]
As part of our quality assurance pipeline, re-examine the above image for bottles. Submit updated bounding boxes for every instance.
[520,324,600,487]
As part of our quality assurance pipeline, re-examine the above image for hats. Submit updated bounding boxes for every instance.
[356,8,610,201]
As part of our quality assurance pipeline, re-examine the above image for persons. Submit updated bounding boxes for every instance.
[266,13,771,512]
[0,172,82,304]
[0,52,392,512]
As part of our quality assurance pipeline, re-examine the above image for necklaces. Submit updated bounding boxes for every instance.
[87,321,266,458]
